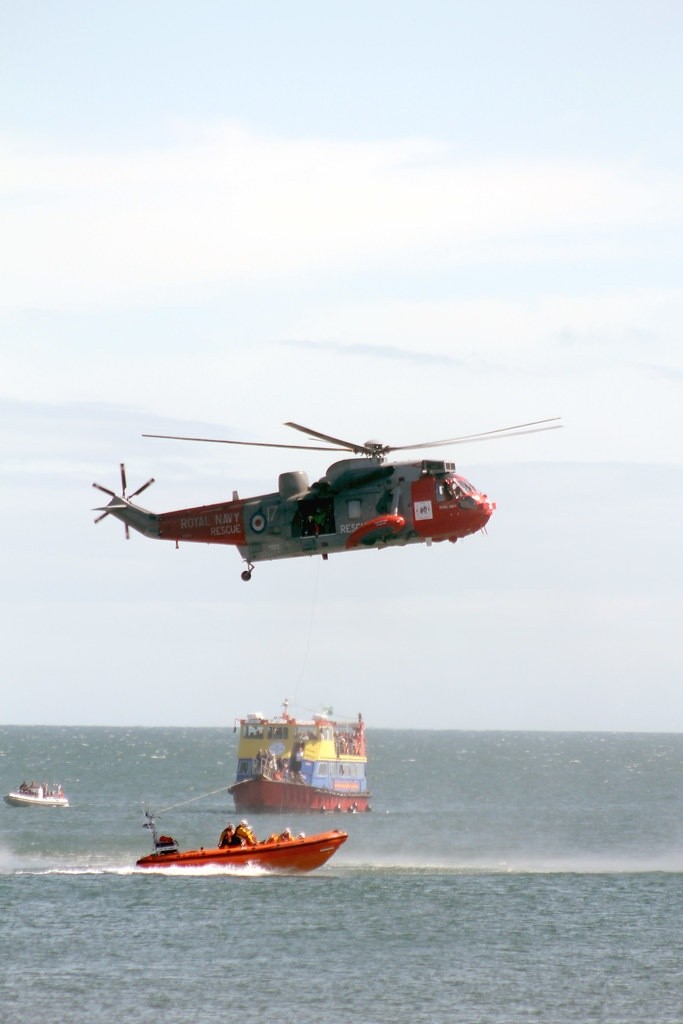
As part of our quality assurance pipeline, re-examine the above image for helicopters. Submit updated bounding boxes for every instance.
[89,414,564,581]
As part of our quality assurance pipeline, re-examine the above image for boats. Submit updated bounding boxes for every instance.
[135,828,349,873]
[228,698,373,814]
[4,791,69,808]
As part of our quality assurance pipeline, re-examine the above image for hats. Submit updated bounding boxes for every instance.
[241,820,248,825]
[229,824,234,829]
[247,826,252,830]
[283,829,291,835]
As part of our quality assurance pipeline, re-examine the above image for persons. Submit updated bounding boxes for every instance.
[301,504,337,539]
[18,781,63,799]
[254,747,306,784]
[249,727,288,737]
[218,820,306,851]
[294,731,360,756]
[442,482,453,501]
